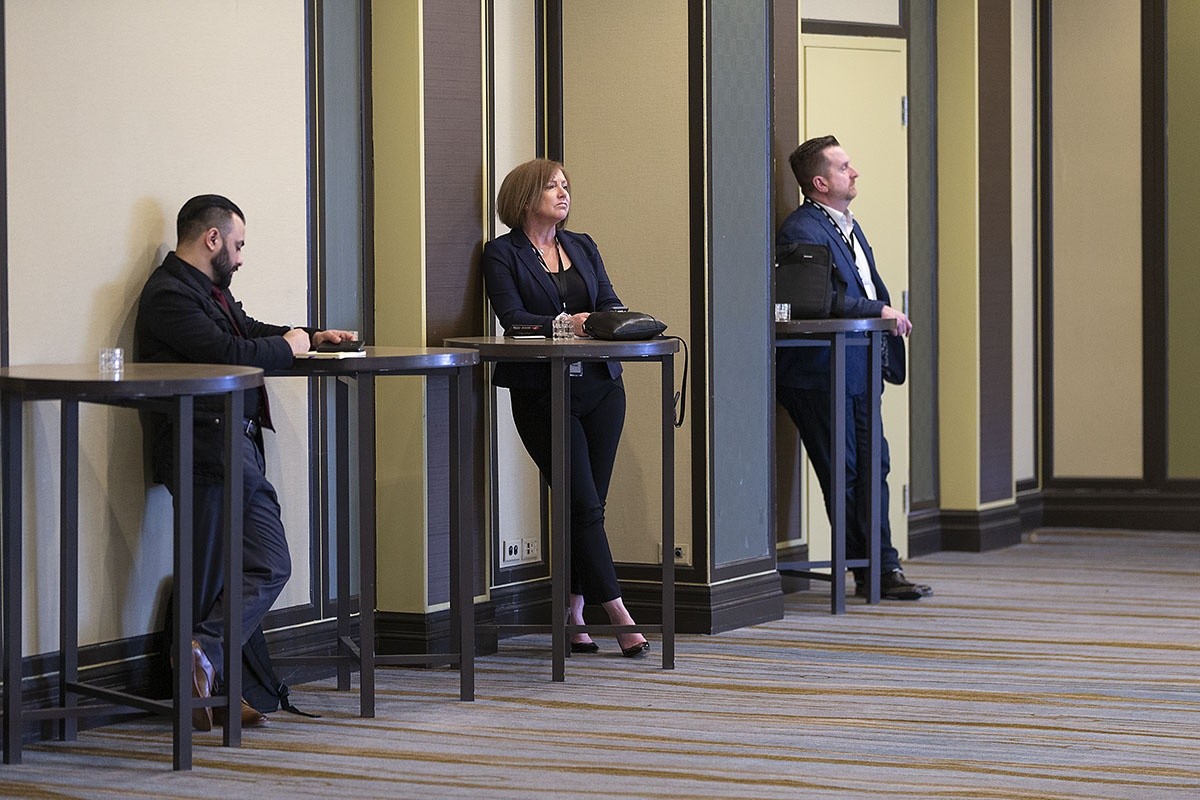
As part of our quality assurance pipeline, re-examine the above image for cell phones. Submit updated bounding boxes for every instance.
[317,340,365,352]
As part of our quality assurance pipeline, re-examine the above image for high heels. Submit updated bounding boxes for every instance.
[616,634,651,655]
[571,641,599,653]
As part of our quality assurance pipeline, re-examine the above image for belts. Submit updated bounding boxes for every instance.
[243,417,261,439]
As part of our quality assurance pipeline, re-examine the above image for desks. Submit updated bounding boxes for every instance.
[0,364,264,769]
[773,317,898,615]
[264,346,481,718]
[446,336,680,684]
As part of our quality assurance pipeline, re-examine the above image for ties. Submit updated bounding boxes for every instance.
[210,285,230,312]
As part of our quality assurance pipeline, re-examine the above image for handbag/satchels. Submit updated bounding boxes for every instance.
[776,243,848,318]
[582,311,667,341]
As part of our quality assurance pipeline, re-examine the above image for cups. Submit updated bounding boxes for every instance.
[347,330,359,342]
[774,304,791,322]
[552,320,575,339]
[99,347,124,374]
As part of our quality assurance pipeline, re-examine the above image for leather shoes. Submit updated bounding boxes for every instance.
[858,580,922,600]
[241,702,268,726]
[894,574,933,598]
[169,638,216,731]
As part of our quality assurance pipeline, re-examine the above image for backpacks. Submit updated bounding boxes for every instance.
[241,624,322,718]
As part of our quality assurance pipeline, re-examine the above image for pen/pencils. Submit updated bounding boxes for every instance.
[903,291,906,315]
[289,321,294,331]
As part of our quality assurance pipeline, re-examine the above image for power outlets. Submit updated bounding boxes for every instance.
[659,542,689,565]
[504,539,522,562]
[523,538,537,558]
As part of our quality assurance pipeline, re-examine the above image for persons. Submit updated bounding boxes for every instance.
[776,135,933,599]
[482,159,651,659]
[136,196,354,733]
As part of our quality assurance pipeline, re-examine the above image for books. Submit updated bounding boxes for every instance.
[296,351,366,359]
